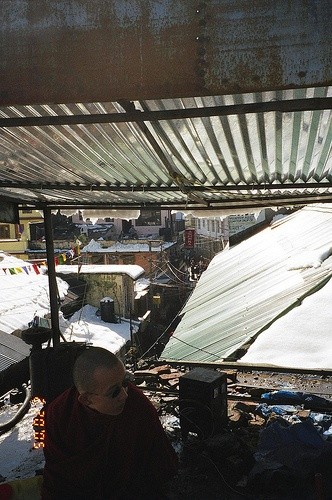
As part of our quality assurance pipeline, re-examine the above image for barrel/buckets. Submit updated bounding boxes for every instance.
[100,301,114,323]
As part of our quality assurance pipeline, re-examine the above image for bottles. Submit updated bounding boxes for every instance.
[314,473,326,500]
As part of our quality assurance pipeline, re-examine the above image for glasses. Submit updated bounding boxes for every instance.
[83,377,131,400]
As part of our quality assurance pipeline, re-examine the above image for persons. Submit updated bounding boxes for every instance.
[44,346,176,500]
